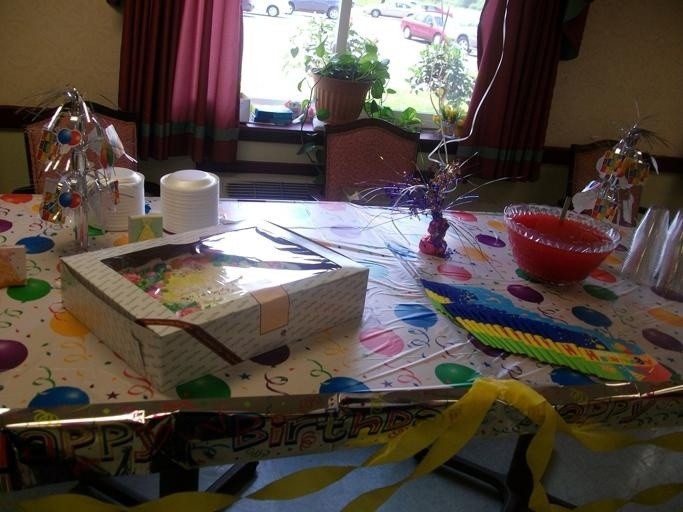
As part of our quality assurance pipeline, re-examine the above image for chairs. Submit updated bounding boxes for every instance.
[556,139,651,228]
[12,100,160,210]
[306,116,425,211]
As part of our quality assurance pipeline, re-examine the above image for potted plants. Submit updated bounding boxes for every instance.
[281,10,428,186]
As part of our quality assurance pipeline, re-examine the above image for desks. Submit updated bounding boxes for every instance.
[3,192,683,512]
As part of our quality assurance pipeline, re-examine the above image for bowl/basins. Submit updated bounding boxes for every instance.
[504,203,622,288]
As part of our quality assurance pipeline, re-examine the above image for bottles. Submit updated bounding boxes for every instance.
[623,205,683,300]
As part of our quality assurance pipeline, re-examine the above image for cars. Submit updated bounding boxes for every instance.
[242,0,355,20]
[363,0,482,53]
